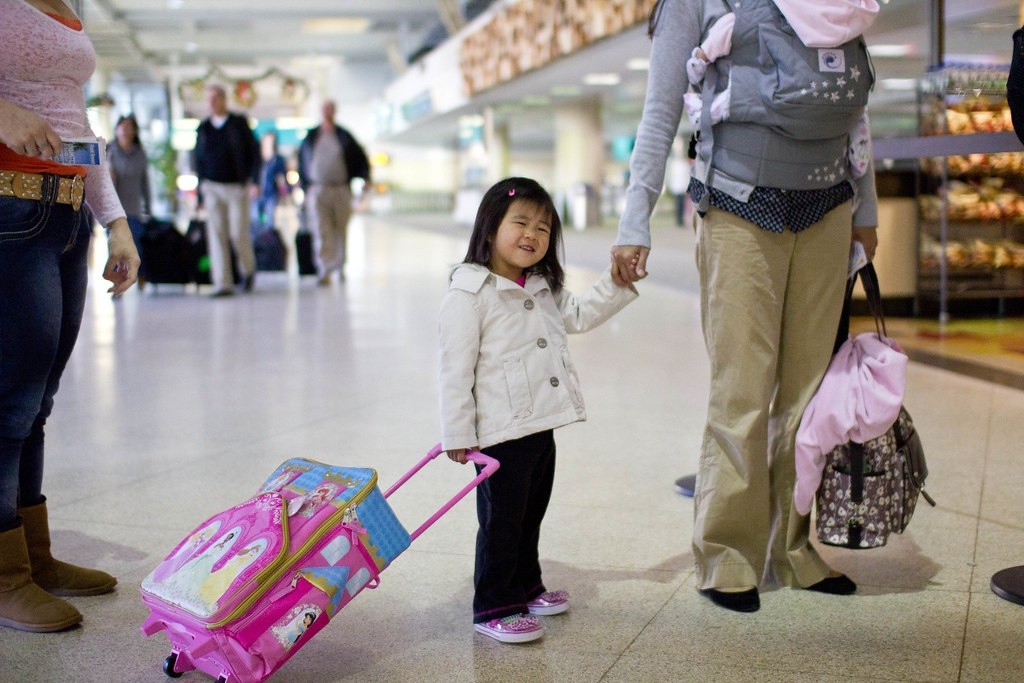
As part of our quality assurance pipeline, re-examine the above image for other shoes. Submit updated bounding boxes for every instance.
[803,574,857,596]
[990,565,1024,606]
[700,587,761,613]
[527,590,570,615]
[474,613,544,643]
[675,473,697,497]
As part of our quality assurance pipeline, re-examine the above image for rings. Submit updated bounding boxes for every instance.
[39,143,49,151]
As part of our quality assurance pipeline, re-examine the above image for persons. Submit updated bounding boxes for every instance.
[990,25,1024,606]
[104,86,372,299]
[610,0,880,612]
[0,0,142,633]
[438,177,649,643]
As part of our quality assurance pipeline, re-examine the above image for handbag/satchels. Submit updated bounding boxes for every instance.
[814,263,936,550]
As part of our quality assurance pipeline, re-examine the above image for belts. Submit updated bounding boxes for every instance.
[0,169,86,211]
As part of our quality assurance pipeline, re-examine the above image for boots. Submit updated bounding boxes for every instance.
[0,521,84,631]
[19,493,118,596]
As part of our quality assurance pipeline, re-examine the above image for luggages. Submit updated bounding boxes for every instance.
[142,219,183,285]
[138,441,501,683]
[186,215,214,284]
[296,229,316,275]
